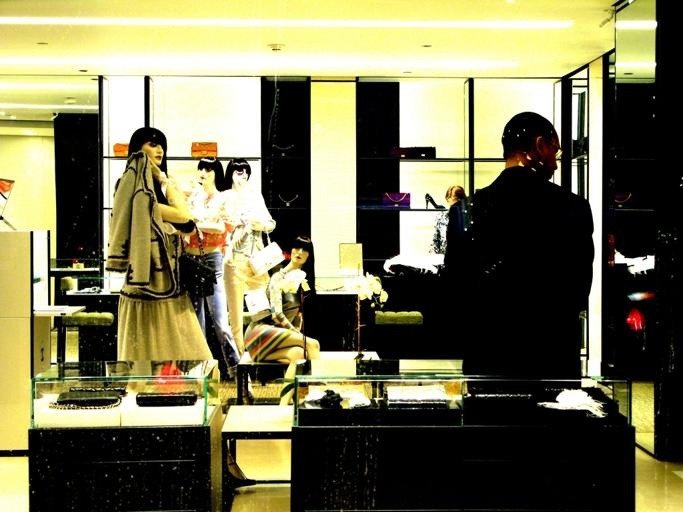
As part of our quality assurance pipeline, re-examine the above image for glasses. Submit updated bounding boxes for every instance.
[544,140,564,160]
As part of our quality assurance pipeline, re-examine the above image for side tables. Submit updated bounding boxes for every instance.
[222,404,293,502]
[301,289,360,351]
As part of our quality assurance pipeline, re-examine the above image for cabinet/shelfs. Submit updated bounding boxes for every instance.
[31,375,225,508]
[2,229,52,453]
[354,74,571,273]
[95,71,312,260]
[292,372,636,511]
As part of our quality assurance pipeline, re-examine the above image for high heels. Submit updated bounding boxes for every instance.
[425,193,446,208]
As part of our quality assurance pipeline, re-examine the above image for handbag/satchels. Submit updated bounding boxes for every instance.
[114,142,130,157]
[386,384,448,407]
[57,381,128,406]
[248,241,286,276]
[190,229,227,248]
[135,392,196,406]
[178,252,217,297]
[463,388,535,406]
[245,286,272,323]
[191,141,217,156]
[383,192,410,208]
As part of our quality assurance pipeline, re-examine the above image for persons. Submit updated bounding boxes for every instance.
[179,155,254,389]
[441,111,596,377]
[104,128,215,362]
[430,185,468,254]
[220,157,277,355]
[243,236,321,376]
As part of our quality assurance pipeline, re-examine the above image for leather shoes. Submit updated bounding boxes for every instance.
[227,464,257,485]
